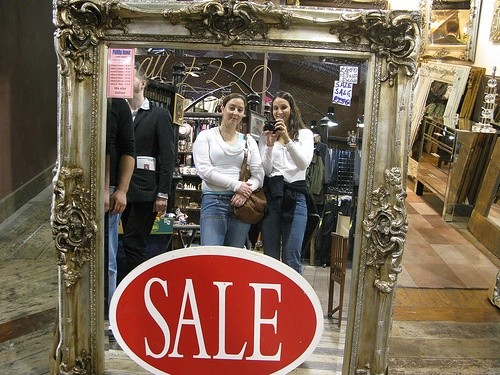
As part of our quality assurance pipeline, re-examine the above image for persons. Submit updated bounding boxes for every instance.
[194,94,266,245]
[259,91,315,275]
[105,97,135,345]
[120,61,178,270]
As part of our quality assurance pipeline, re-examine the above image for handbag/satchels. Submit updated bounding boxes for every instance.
[233,134,268,224]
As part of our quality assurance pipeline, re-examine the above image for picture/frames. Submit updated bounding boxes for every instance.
[249,109,268,141]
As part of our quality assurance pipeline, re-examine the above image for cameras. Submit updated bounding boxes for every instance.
[263,120,282,134]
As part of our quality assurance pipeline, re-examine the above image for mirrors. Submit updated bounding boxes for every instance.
[486,182,500,228]
[408,81,453,161]
[103,46,370,375]
[429,0,472,45]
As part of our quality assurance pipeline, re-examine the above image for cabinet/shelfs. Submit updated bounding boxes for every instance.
[144,77,249,245]
[414,114,494,223]
